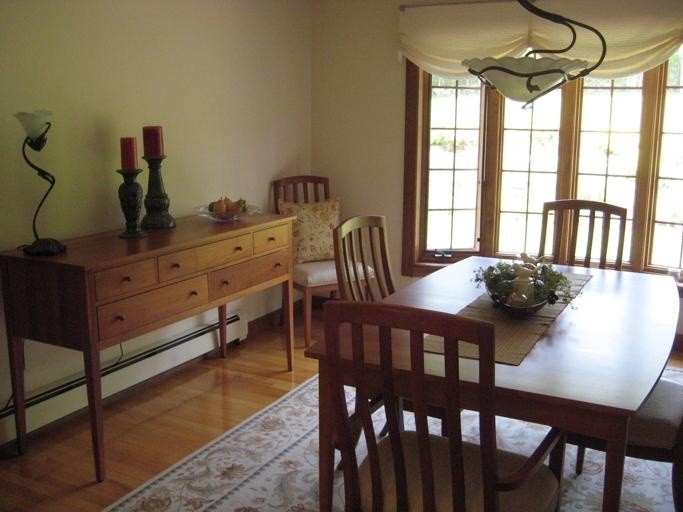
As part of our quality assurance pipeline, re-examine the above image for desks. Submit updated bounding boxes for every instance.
[305,256,680,512]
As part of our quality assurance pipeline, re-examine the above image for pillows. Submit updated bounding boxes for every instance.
[278,199,343,263]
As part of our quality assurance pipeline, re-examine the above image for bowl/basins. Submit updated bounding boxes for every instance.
[193,203,261,224]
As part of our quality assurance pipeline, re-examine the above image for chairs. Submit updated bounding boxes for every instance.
[538,200,627,270]
[549,379,683,512]
[272,176,375,348]
[317,300,566,512]
[334,213,465,476]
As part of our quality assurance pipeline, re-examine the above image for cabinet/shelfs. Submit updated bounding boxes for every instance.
[1,208,298,483]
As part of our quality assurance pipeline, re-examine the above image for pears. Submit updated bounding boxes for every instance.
[208,195,246,216]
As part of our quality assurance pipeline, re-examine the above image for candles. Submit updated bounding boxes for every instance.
[120,136,138,172]
[143,124,164,156]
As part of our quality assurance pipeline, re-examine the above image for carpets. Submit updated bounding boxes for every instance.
[104,367,683,512]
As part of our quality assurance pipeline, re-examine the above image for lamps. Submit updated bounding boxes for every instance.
[463,0,605,109]
[14,108,65,257]
